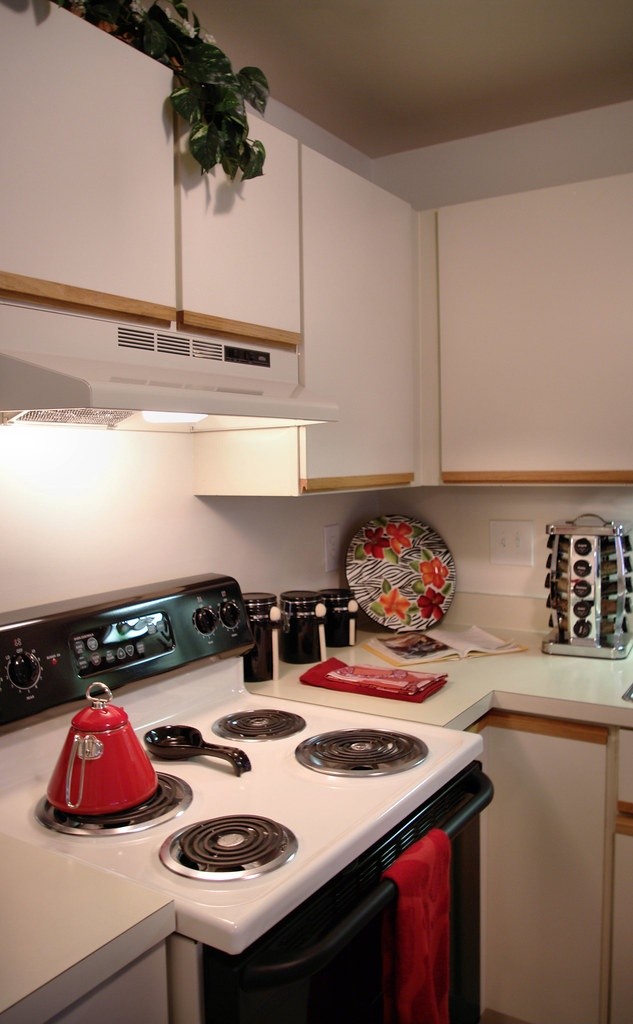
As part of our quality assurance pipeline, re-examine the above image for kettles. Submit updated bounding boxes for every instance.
[46,681,156,815]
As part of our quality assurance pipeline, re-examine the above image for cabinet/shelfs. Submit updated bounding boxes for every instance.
[0,0,301,347]
[192,144,423,497]
[464,712,633,1024]
[429,171,632,489]
[47,941,169,1024]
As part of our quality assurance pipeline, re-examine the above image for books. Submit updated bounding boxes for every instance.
[361,625,529,668]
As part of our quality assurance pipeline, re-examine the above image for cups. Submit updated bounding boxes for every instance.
[320,589,358,647]
[239,592,281,683]
[281,590,328,664]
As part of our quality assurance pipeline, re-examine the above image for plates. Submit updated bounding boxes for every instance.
[347,517,456,632]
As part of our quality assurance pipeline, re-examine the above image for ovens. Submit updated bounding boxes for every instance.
[168,758,493,1024]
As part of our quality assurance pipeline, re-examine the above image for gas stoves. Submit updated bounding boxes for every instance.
[0,571,483,956]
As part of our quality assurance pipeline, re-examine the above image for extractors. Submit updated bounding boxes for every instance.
[0,302,337,433]
